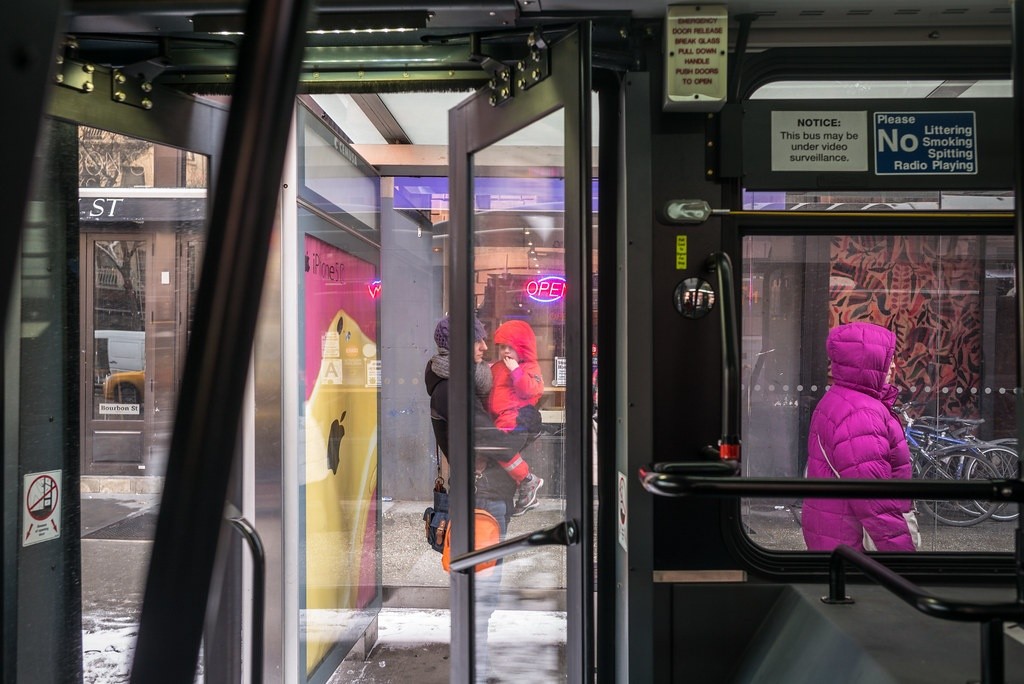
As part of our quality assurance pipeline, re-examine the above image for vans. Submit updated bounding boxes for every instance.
[93,329,146,388]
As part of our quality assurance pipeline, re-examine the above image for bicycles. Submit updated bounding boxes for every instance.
[892,401,1022,526]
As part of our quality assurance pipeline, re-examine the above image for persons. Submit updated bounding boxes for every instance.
[800,321,923,552]
[425,311,542,684]
[482,320,544,516]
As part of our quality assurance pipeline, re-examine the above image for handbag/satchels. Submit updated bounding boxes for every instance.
[862,512,922,551]
[441,509,500,577]
[423,483,448,554]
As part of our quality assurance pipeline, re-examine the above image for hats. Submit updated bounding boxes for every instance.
[434,314,486,351]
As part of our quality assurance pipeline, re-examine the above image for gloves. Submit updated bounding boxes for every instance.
[516,405,542,440]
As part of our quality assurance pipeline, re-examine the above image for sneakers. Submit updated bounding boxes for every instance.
[511,497,539,516]
[518,473,544,507]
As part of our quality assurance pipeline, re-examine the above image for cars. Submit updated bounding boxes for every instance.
[102,370,146,409]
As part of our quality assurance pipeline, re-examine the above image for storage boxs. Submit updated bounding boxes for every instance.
[523,362,554,386]
[493,286,554,326]
[500,328,556,361]
